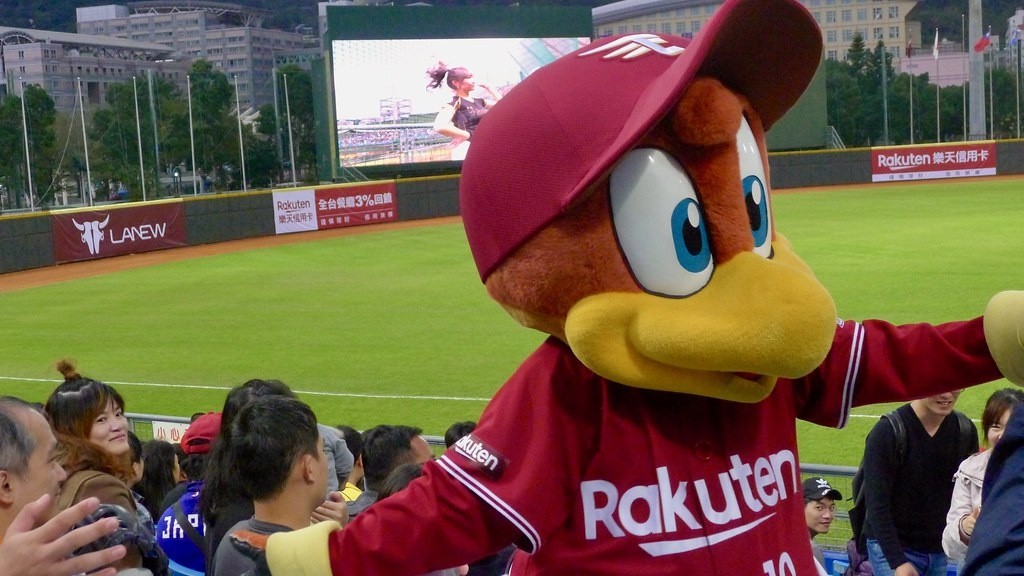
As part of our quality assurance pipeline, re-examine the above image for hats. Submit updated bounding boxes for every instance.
[181,410,222,452]
[801,477,843,501]
[459,0,826,284]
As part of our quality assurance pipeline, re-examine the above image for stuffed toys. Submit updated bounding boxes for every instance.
[229,2,1024,575]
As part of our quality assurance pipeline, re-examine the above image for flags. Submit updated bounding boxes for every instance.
[933,30,939,61]
[905,36,912,58]
[974,30,991,52]
[1010,23,1021,47]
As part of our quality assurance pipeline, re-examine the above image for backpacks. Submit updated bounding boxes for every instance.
[849,411,973,538]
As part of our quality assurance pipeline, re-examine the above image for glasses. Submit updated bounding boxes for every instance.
[951,388,965,393]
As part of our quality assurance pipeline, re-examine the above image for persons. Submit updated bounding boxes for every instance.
[426,60,506,160]
[0,357,516,575]
[801,380,1024,576]
